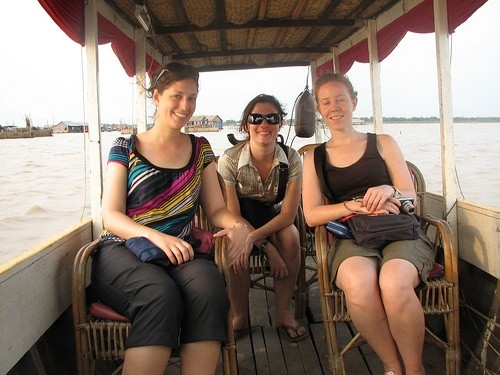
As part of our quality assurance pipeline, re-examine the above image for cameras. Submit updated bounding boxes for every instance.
[396,197,416,215]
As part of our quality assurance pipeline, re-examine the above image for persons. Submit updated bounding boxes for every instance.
[90,62,250,375]
[301,73,431,375]
[217,95,308,342]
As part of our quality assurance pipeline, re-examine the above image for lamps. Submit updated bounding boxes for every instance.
[134,4,151,32]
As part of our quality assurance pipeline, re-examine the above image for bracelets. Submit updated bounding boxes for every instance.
[392,186,401,198]
[344,201,355,212]
[260,240,269,251]
[232,220,247,228]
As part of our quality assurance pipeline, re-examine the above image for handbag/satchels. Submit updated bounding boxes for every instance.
[348,214,420,249]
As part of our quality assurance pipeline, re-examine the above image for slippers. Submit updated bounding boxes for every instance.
[233,325,249,341]
[279,321,309,342]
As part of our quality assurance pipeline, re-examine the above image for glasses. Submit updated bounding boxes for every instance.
[246,114,280,126]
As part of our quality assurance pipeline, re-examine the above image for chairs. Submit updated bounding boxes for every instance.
[294,143,325,319]
[313,160,462,375]
[71,170,241,375]
[216,200,305,320]
